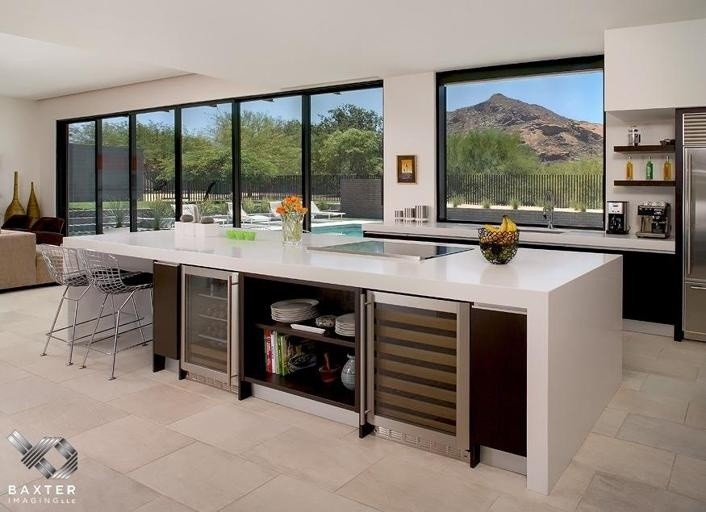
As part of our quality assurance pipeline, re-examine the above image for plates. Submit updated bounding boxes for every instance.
[334,313,355,337]
[270,298,320,324]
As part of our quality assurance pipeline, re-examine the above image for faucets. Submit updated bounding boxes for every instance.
[543,189,554,228]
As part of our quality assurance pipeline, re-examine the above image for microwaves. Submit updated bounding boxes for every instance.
[627,122,640,146]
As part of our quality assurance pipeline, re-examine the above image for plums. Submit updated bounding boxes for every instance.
[492,246,501,254]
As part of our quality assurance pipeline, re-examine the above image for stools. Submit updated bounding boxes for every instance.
[36,243,63,287]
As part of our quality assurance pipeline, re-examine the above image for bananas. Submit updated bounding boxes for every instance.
[482,214,517,245]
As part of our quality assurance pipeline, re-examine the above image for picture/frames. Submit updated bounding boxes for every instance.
[395,153,417,185]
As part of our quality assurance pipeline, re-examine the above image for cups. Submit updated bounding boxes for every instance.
[319,364,339,382]
[227,229,254,241]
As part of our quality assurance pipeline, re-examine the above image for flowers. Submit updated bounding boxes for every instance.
[276,194,308,241]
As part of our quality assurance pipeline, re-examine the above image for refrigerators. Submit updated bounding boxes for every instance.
[682,111,706,344]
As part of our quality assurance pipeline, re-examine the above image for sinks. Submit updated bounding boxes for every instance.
[519,228,566,234]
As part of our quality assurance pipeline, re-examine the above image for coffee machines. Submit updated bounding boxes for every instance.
[605,200,628,234]
[634,202,672,238]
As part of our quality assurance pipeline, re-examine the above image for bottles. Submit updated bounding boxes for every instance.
[626,155,633,180]
[645,156,654,180]
[4,171,25,222]
[340,353,355,391]
[663,155,672,181]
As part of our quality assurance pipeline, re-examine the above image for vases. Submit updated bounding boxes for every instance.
[279,212,303,248]
[3,170,28,224]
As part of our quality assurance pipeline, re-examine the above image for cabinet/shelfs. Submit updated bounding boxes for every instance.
[239,273,362,438]
[359,285,483,470]
[611,144,676,188]
[177,261,240,398]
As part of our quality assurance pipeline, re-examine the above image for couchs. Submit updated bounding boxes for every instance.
[0,228,36,293]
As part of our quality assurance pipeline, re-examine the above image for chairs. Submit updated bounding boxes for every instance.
[0,214,32,231]
[170,202,195,217]
[224,201,270,226]
[299,198,346,221]
[266,201,282,222]
[77,247,154,381]
[33,240,148,369]
[16,216,65,246]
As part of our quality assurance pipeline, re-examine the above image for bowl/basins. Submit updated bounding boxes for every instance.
[315,315,335,327]
